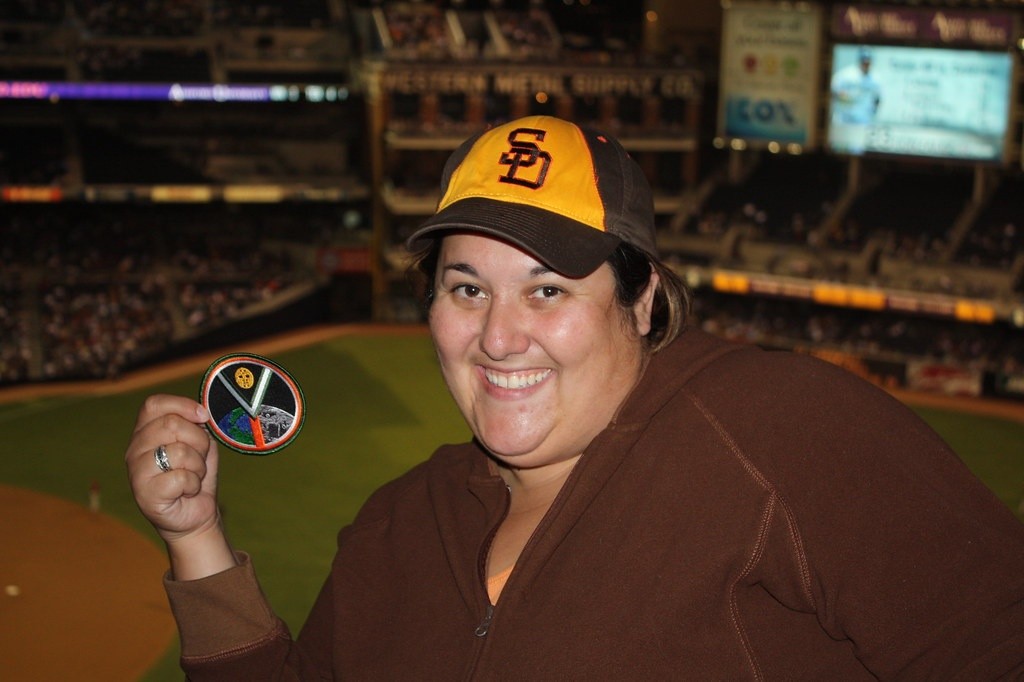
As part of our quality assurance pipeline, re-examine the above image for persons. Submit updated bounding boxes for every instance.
[123,111,1024,682]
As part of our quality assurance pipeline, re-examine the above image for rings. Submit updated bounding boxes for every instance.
[154,444,174,474]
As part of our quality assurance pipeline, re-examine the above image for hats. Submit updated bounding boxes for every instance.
[406,114,661,279]
